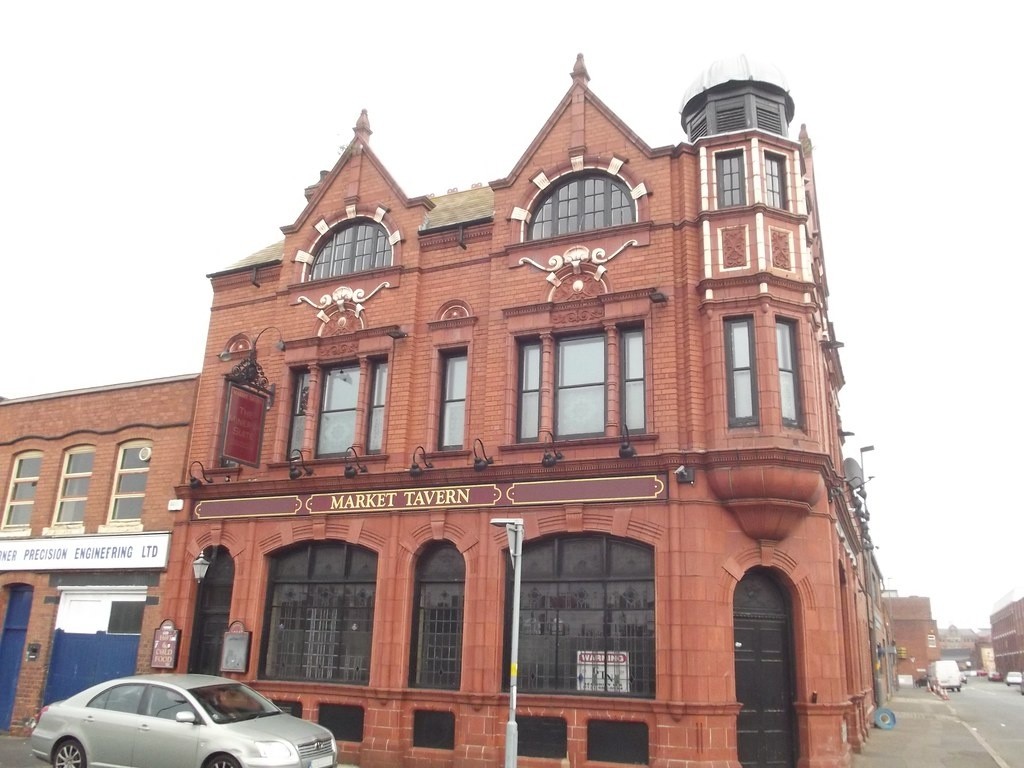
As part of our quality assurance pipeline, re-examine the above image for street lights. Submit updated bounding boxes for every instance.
[491,518,526,768]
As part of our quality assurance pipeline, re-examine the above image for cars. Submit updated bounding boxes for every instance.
[915,674,929,688]
[28,673,340,768]
[988,673,993,681]
[977,670,988,676]
[1020,671,1024,696]
[1005,671,1022,687]
[960,672,967,685]
[990,673,1003,682]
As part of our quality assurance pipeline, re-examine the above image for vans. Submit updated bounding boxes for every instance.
[928,660,962,693]
